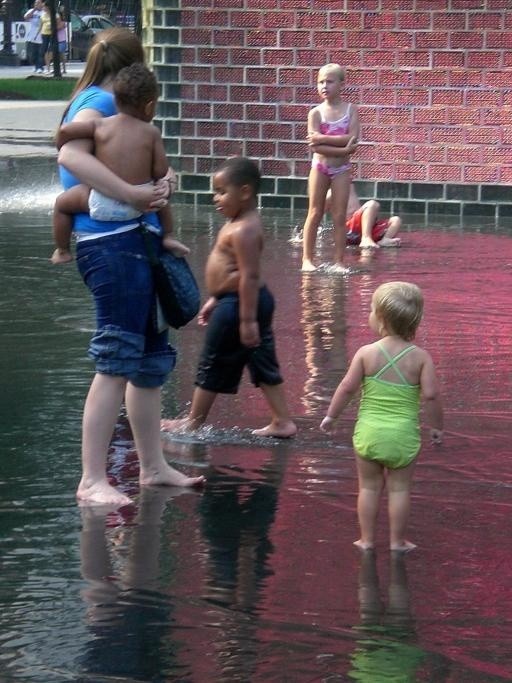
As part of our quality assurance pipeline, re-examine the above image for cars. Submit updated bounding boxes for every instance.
[72,13,117,62]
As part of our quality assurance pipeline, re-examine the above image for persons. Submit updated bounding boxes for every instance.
[77,484,198,683]
[196,445,286,681]
[24,0,43,73]
[321,282,444,550]
[33,1,60,75]
[300,62,359,271]
[347,549,458,683]
[55,29,203,508]
[47,63,190,264]
[322,178,402,249]
[43,11,66,75]
[159,156,301,439]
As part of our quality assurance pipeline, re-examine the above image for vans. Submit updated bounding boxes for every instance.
[116,15,134,26]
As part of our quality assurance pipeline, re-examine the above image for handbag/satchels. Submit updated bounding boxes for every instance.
[150,256,203,326]
